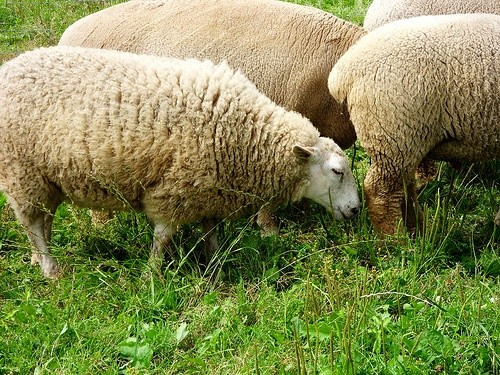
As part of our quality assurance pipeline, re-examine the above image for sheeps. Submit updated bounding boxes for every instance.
[0,46,362,284]
[52,0,500,247]
